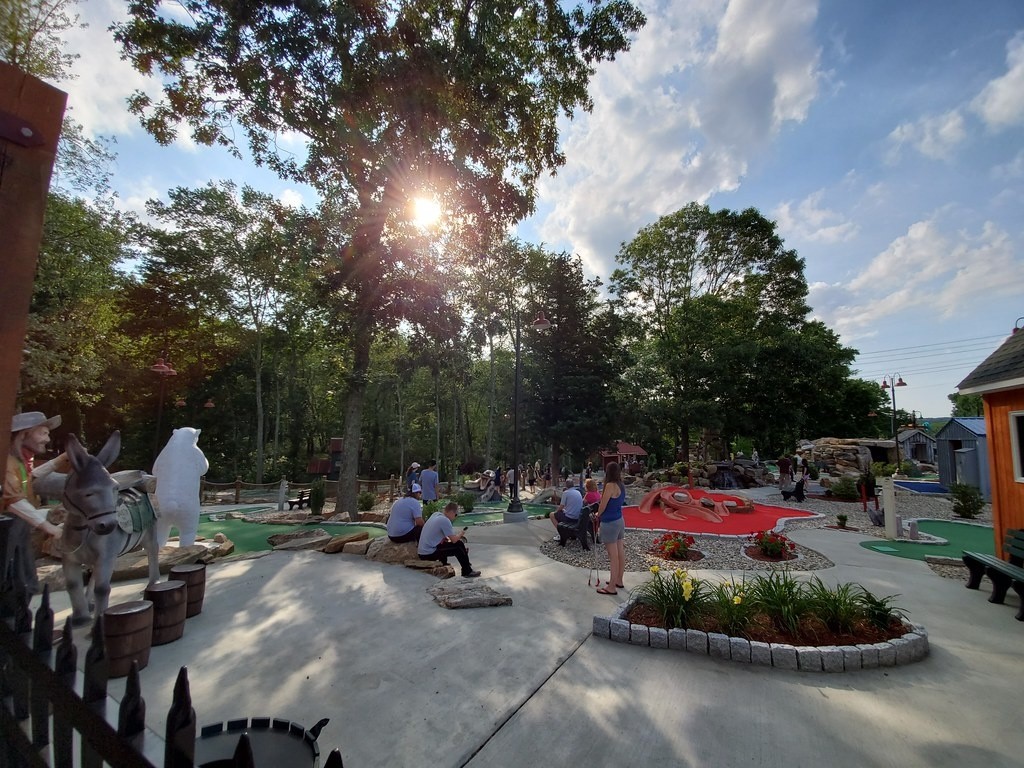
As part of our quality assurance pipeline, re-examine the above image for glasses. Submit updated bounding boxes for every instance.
[453,512,457,517]
[415,492,422,494]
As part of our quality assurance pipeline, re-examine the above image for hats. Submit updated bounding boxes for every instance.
[407,483,421,492]
[412,462,420,468]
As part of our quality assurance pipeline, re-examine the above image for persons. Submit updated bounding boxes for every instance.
[776,454,797,489]
[405,459,439,513]
[802,459,810,490]
[549,479,583,541]
[494,458,551,499]
[585,462,593,478]
[752,447,759,466]
[582,479,603,533]
[417,502,481,578]
[387,483,425,544]
[0,411,64,698]
[736,449,744,456]
[594,462,626,595]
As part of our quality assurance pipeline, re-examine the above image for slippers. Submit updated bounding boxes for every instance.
[597,588,617,595]
[606,581,624,588]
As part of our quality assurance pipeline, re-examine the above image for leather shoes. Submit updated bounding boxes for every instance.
[464,570,481,578]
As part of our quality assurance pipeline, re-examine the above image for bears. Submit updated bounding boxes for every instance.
[152,426,210,546]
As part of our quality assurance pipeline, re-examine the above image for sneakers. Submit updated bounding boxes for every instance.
[553,535,561,540]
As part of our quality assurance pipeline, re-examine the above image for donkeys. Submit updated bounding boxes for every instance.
[59,429,161,634]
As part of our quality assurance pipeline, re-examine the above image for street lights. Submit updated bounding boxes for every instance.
[508,310,551,512]
[175,399,215,428]
[882,372,907,475]
[149,358,178,475]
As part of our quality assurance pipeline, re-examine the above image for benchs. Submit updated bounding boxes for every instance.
[557,500,601,550]
[961,528,1024,621]
[287,489,311,510]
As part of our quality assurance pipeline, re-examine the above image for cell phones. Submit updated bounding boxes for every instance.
[463,527,468,531]
